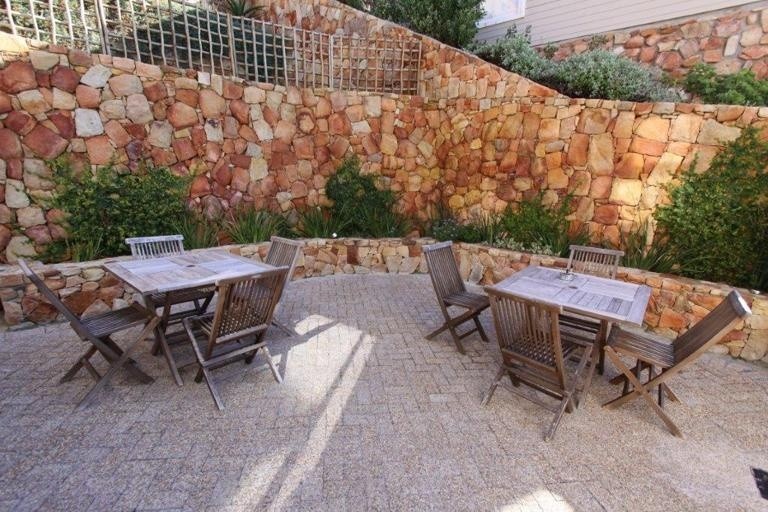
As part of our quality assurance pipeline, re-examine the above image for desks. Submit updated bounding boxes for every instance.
[100,247,287,387]
[485,264,652,408]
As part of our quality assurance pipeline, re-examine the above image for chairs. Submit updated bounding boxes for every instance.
[124,233,222,357]
[228,234,304,345]
[485,285,594,444]
[180,264,291,410]
[16,257,162,411]
[604,286,752,441]
[556,245,627,377]
[423,241,492,355]
